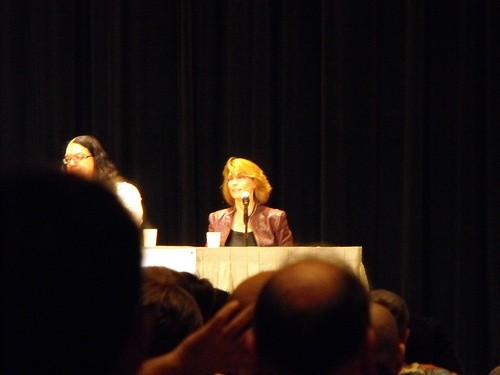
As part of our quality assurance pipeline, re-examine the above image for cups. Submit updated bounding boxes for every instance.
[207,232,221,247]
[143,229,158,247]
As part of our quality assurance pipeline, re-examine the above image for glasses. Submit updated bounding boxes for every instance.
[63,154,93,165]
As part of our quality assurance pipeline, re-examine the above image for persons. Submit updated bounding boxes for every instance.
[205,155,294,246]
[63,135,143,226]
[0,163,500,374]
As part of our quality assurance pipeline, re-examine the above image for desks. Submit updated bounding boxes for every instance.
[140,246,369,295]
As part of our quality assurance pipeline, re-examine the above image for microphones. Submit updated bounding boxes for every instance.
[241,191,250,206]
